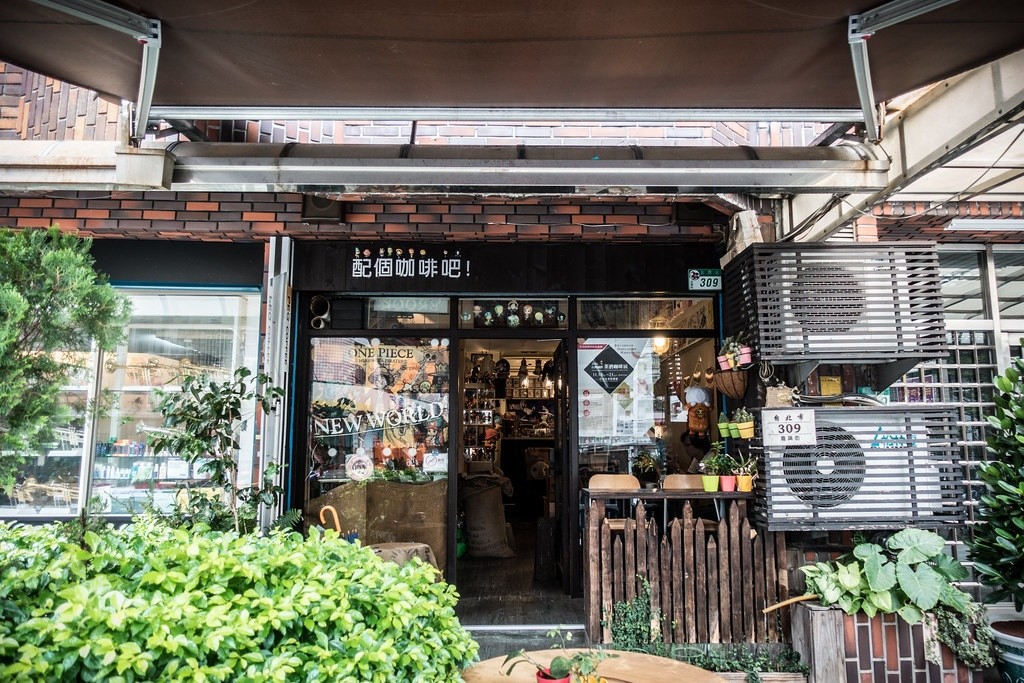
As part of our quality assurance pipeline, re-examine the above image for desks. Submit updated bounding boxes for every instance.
[582,488,761,530]
[461,648,724,683]
[317,469,378,494]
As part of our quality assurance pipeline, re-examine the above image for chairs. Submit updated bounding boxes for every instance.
[663,474,721,540]
[589,474,653,531]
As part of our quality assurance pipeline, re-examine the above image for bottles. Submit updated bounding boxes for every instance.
[100,465,131,480]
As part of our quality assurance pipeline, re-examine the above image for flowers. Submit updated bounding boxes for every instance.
[698,439,726,475]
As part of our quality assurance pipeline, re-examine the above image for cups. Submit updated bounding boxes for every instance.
[115,439,152,455]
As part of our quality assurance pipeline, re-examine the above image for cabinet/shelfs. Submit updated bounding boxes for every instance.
[3,383,237,516]
[462,370,559,470]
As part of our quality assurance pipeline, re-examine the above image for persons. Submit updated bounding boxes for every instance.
[674,429,716,474]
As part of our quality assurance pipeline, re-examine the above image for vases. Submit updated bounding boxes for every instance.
[701,475,718,492]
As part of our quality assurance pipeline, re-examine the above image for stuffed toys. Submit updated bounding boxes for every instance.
[683,384,713,439]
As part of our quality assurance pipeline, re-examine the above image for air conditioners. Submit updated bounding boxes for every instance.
[763,248,915,360]
[766,414,943,527]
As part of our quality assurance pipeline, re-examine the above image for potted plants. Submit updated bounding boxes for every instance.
[717,343,755,497]
[545,624,618,683]
[956,336,1024,683]
[499,649,571,683]
